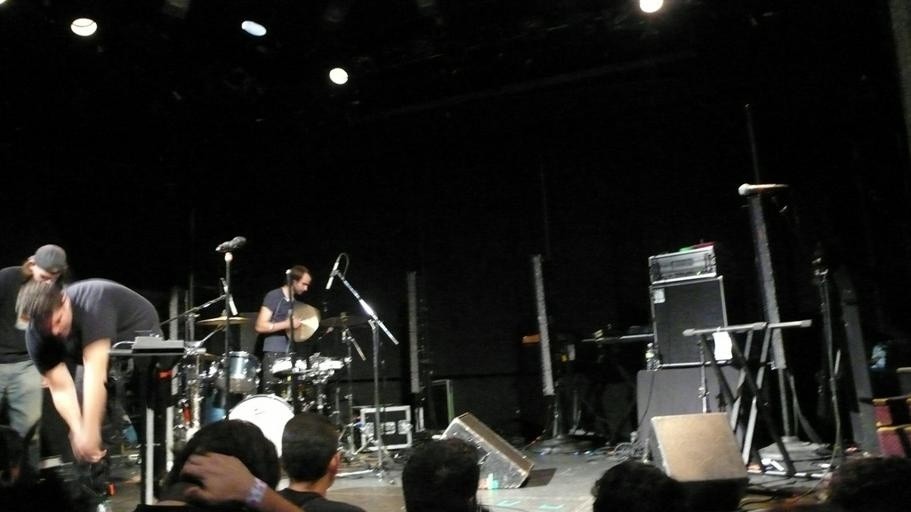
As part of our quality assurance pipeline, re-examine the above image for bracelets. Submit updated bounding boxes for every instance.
[246,479,268,508]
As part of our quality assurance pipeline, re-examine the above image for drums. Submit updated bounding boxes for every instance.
[311,356,340,380]
[226,393,296,458]
[269,355,307,378]
[216,351,262,393]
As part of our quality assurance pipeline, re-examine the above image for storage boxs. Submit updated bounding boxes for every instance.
[648,245,729,285]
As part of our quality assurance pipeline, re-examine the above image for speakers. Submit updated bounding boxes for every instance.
[437,409,536,490]
[650,273,737,366]
[647,409,752,488]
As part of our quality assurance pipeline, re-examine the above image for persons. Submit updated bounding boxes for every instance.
[591,460,686,512]
[0,244,68,475]
[15,277,163,496]
[254,265,312,400]
[402,438,487,512]
[825,456,911,512]
[276,412,365,512]
[2,466,105,512]
[133,420,305,512]
[0,424,29,490]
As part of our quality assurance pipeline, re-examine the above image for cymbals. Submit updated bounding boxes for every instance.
[200,353,215,360]
[196,316,249,326]
[285,304,321,342]
[322,315,358,328]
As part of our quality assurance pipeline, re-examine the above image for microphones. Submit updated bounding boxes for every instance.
[211,231,249,255]
[735,182,794,196]
[326,260,340,291]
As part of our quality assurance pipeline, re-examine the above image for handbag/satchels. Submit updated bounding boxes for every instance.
[26,456,110,511]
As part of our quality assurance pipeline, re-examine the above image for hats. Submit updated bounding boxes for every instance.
[36,245,67,273]
